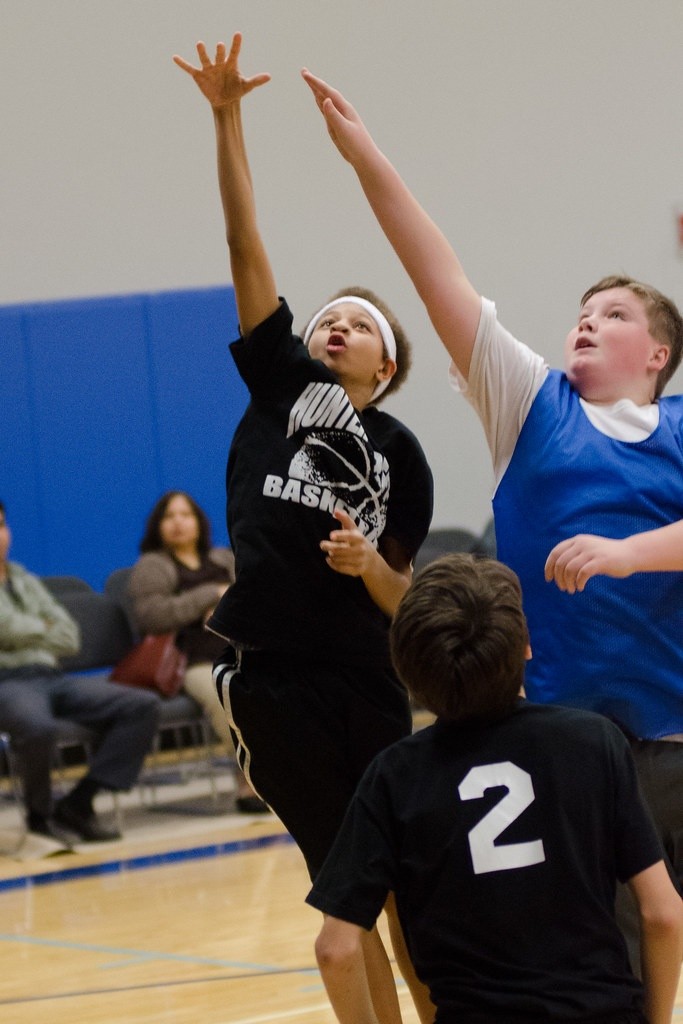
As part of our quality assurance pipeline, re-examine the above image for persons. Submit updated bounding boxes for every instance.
[173,33,437,1024]
[303,552,683,1024]
[297,67,683,896]
[0,498,161,856]
[126,489,272,814]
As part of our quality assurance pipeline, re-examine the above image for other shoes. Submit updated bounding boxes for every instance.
[235,794,270,813]
[51,798,122,843]
[24,814,75,851]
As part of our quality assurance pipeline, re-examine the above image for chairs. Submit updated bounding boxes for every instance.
[412,519,496,578]
[0,567,234,834]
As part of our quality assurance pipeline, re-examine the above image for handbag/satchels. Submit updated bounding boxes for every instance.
[106,629,188,697]
